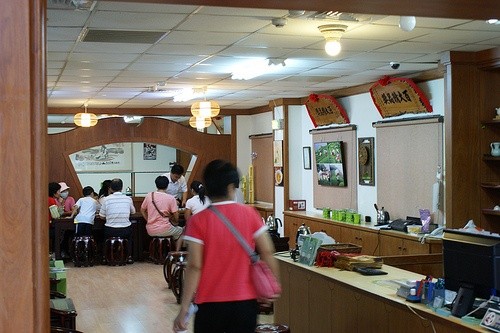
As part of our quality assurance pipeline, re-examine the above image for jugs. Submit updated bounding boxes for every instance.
[490,143,500,156]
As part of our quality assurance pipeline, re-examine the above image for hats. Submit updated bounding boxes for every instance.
[58,182,70,192]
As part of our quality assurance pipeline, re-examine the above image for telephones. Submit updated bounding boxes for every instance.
[380,214,415,233]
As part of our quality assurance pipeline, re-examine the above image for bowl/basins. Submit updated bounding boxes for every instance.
[407,225,422,234]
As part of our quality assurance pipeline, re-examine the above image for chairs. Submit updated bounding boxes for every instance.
[49,277,78,333]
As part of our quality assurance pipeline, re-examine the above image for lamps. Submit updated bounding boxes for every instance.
[189,89,221,131]
[318,25,348,54]
[74,102,98,127]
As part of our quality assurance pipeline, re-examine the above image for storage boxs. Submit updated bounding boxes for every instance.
[50,260,66,294]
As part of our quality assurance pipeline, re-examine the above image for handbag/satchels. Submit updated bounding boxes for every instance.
[249,261,280,299]
[169,216,178,226]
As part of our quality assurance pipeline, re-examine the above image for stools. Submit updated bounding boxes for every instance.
[163,249,190,290]
[73,235,97,268]
[104,236,129,266]
[171,261,189,302]
[149,237,175,265]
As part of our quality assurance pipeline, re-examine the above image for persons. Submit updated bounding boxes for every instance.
[140,176,184,262]
[65,186,101,262]
[172,160,281,333]
[98,179,136,263]
[48,182,75,260]
[184,182,211,221]
[161,165,188,206]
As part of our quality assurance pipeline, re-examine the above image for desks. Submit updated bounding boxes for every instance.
[54,214,148,263]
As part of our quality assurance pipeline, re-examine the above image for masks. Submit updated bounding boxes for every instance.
[60,191,69,199]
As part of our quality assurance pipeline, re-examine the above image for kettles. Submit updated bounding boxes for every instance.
[261,213,282,233]
[377,207,390,225]
[295,223,311,255]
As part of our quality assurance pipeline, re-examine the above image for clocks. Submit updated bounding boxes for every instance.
[358,137,375,185]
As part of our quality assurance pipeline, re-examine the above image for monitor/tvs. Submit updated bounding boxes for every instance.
[314,141,347,187]
[442,228,500,318]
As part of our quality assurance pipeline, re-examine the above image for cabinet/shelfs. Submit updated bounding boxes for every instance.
[256,204,273,220]
[440,46,500,234]
[283,213,442,256]
[272,252,496,333]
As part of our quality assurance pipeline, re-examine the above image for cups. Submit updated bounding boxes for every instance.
[415,282,434,304]
[323,208,360,224]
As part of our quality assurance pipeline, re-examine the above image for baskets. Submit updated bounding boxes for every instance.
[319,244,361,254]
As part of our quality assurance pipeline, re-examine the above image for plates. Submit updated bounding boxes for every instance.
[359,146,368,165]
[275,170,283,184]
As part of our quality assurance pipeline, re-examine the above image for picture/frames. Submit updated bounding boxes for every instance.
[303,147,312,169]
